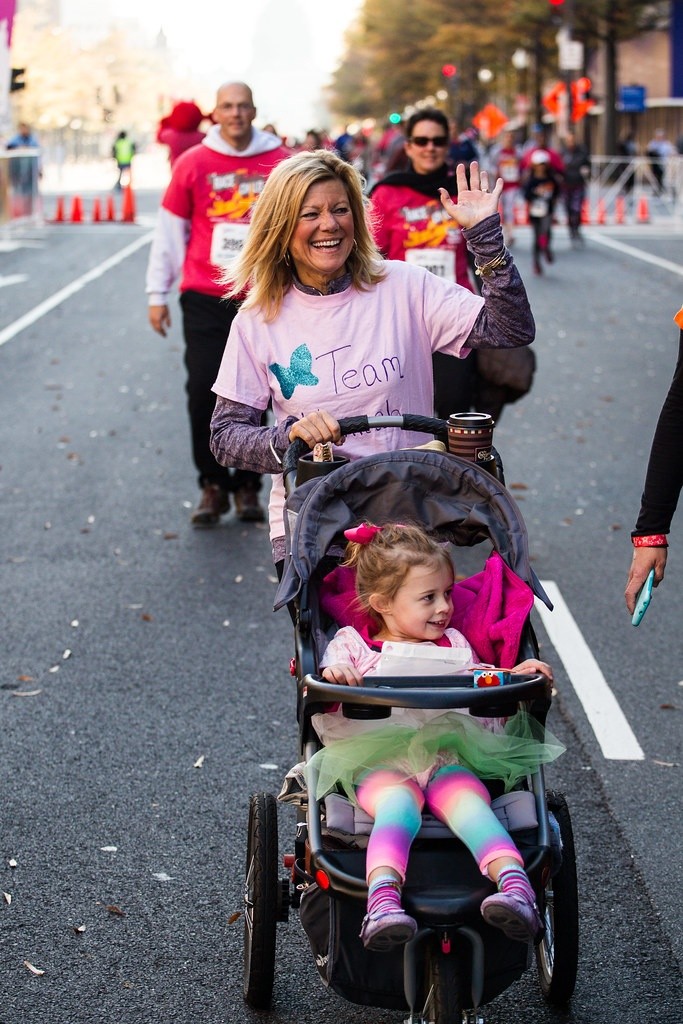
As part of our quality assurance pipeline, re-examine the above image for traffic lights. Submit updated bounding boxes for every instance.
[9,68,28,94]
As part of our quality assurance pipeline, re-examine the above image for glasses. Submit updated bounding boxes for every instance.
[409,135,448,147]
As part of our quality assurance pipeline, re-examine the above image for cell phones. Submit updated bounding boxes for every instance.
[632,569,655,627]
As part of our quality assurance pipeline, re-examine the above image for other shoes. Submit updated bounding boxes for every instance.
[481,891,543,940]
[359,902,418,951]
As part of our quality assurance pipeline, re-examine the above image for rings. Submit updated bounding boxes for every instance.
[482,189,490,193]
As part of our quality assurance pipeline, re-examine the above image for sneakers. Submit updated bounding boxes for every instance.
[192,486,229,525]
[234,491,263,521]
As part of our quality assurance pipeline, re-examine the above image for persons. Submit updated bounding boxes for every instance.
[264,120,481,190]
[368,106,483,300]
[614,127,683,199]
[113,132,135,189]
[314,522,538,950]
[488,126,590,274]
[206,149,536,627]
[6,122,42,197]
[623,305,683,614]
[145,80,300,525]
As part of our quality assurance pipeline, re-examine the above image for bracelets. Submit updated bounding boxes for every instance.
[473,244,507,276]
[633,534,667,547]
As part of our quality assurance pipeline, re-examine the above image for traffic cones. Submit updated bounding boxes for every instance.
[122,185,139,222]
[513,195,659,228]
[72,196,82,222]
[92,197,104,222]
[105,197,117,221]
[54,196,64,222]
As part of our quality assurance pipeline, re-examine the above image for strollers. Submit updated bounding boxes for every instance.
[225,409,579,1008]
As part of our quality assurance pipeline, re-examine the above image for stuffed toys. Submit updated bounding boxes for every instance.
[156,101,215,166]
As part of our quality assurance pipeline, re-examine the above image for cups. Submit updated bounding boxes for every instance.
[445,413,495,463]
